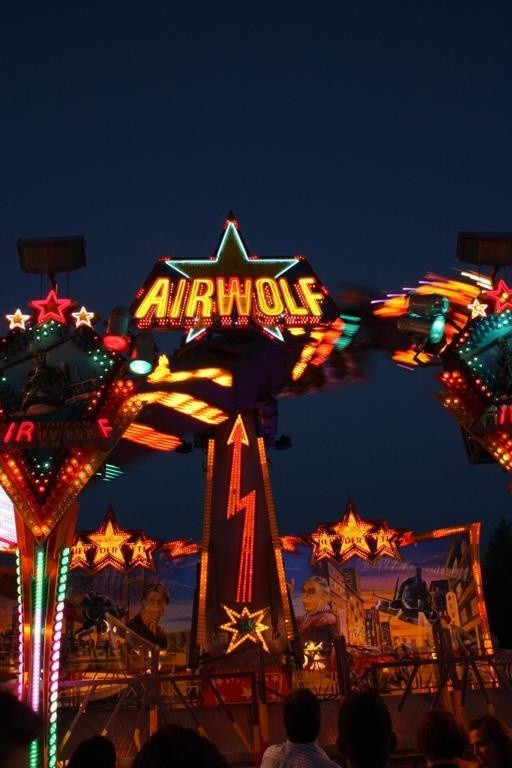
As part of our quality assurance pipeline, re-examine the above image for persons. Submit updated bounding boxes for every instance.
[287,573,336,640]
[128,582,171,649]
